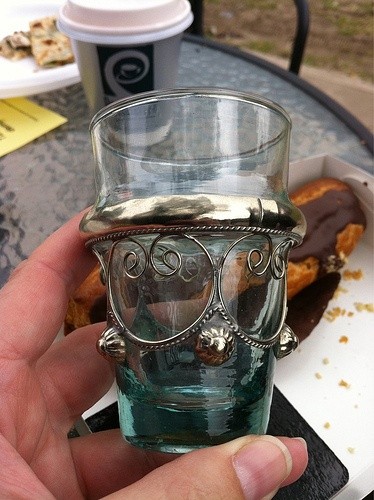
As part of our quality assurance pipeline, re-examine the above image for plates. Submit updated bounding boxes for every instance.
[1,0,80,99]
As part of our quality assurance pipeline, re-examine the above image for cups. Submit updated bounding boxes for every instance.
[79,87,306,455]
[55,0,194,117]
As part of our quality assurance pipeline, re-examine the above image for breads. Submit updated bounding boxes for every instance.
[63,179,369,330]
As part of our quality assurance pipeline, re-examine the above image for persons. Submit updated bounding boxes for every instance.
[0,193,312,500]
